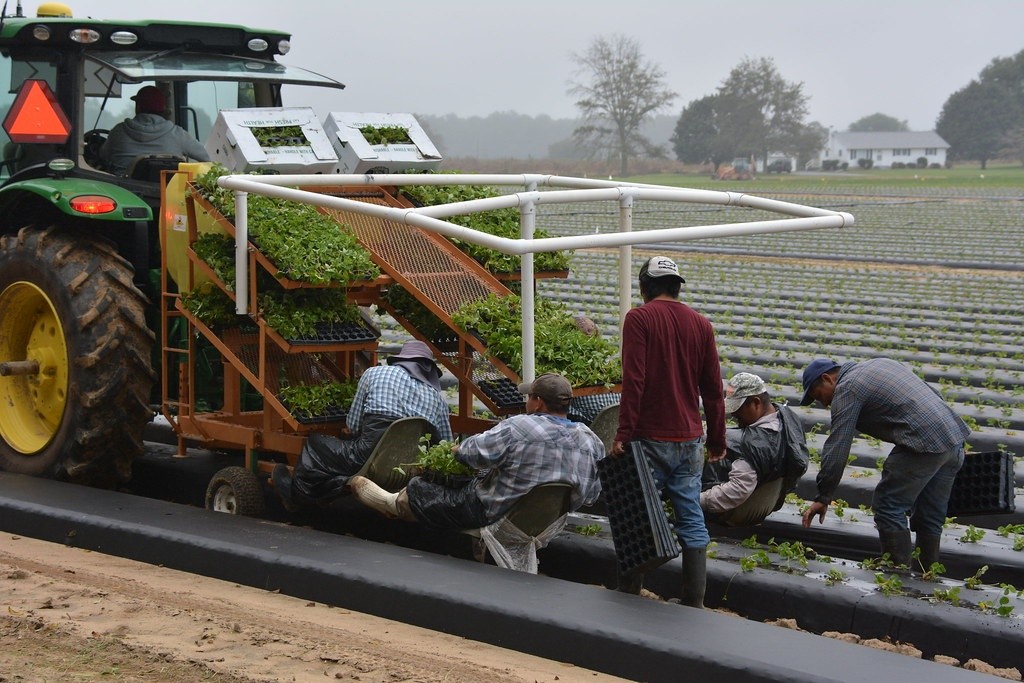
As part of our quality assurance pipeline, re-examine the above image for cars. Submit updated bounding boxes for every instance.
[767,159,792,174]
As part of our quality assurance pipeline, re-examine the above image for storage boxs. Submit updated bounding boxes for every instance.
[204,106,339,174]
[322,111,443,174]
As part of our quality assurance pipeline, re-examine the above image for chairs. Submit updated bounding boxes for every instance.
[327,416,442,492]
[125,153,186,183]
[707,476,784,530]
[455,481,573,577]
[590,403,620,455]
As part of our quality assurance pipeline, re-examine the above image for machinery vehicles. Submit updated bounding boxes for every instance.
[0,0,855,516]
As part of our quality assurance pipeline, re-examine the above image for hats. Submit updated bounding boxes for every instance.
[387,339,443,393]
[518,373,574,405]
[723,372,767,414]
[130,86,164,112]
[798,358,841,407]
[639,255,686,284]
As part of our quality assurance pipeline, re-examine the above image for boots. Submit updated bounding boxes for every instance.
[272,462,313,517]
[913,533,941,574]
[681,547,707,609]
[611,560,644,597]
[351,476,399,522]
[876,527,913,570]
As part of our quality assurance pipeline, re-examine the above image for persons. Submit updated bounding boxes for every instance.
[272,339,454,528]
[800,357,971,577]
[612,256,726,608]
[352,371,608,532]
[97,87,211,178]
[698,373,810,538]
